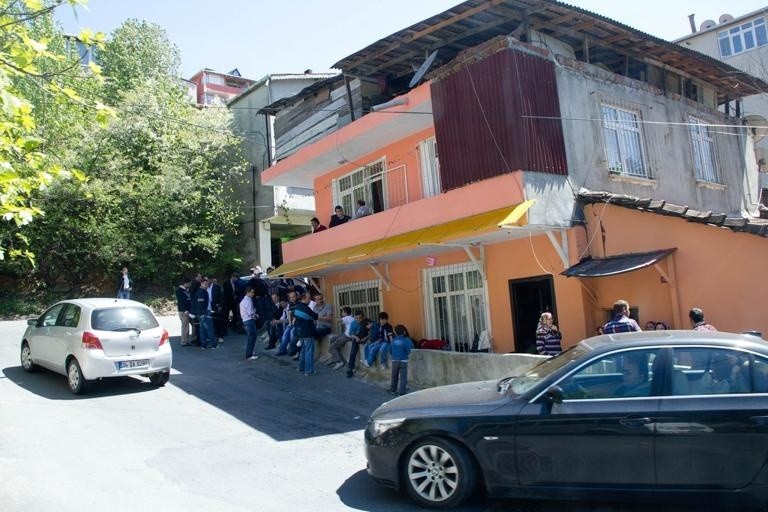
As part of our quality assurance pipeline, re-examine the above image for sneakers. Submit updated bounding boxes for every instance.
[386,389,396,395]
[182,337,224,350]
[360,360,369,368]
[305,370,317,376]
[381,363,385,369]
[296,367,303,371]
[265,346,276,349]
[333,361,344,370]
[276,352,287,355]
[249,355,259,359]
[348,369,353,378]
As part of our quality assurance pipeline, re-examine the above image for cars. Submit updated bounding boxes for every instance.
[361,331,768,504]
[18,296,174,397]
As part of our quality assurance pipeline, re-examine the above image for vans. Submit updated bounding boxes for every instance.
[225,276,315,332]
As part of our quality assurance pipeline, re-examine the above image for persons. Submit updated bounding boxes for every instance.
[535,312,562,357]
[118,267,134,298]
[602,299,642,332]
[348,200,372,221]
[689,308,719,332]
[328,206,352,228]
[311,217,326,232]
[586,353,652,398]
[655,322,668,331]
[644,321,655,331]
[176,265,413,396]
